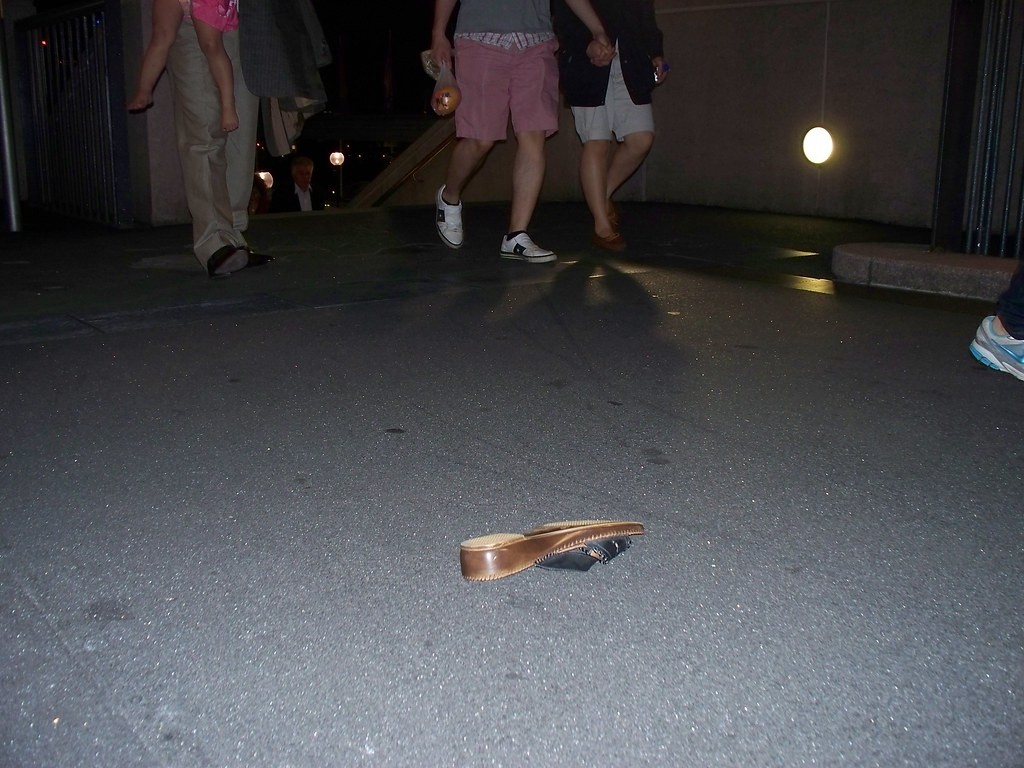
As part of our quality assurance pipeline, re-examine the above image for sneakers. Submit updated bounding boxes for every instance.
[969,316,1024,381]
[499,232,558,263]
[435,183,465,249]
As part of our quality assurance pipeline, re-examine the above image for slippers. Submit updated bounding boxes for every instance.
[459,519,645,581]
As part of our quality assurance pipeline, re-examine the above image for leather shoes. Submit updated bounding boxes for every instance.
[207,244,276,280]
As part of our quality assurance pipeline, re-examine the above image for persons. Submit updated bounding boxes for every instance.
[127,0,240,133]
[431,0,611,263]
[271,157,325,213]
[166,0,329,279]
[248,174,268,214]
[550,0,670,252]
[969,255,1024,381]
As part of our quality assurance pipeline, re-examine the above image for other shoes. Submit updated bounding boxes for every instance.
[592,231,626,252]
[606,202,620,227]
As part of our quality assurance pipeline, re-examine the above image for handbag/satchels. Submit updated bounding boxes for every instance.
[421,46,462,116]
